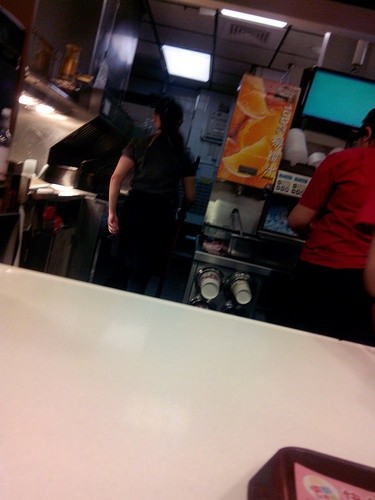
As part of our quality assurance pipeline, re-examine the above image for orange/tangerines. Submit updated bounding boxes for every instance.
[222,76,284,178]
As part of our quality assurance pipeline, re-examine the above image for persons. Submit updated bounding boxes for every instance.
[286,108,375,348]
[100,95,196,302]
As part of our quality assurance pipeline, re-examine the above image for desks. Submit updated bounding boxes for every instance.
[0,262,375,500]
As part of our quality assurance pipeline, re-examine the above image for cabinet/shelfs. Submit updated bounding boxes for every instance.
[182,234,272,318]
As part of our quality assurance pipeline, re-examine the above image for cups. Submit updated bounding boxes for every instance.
[232,280,253,305]
[199,272,221,299]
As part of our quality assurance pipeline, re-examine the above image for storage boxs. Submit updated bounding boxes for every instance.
[247,446,375,500]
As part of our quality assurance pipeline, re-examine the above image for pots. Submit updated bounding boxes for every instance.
[43,165,94,190]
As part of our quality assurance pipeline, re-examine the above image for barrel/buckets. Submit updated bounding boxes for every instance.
[227,233,260,263]
